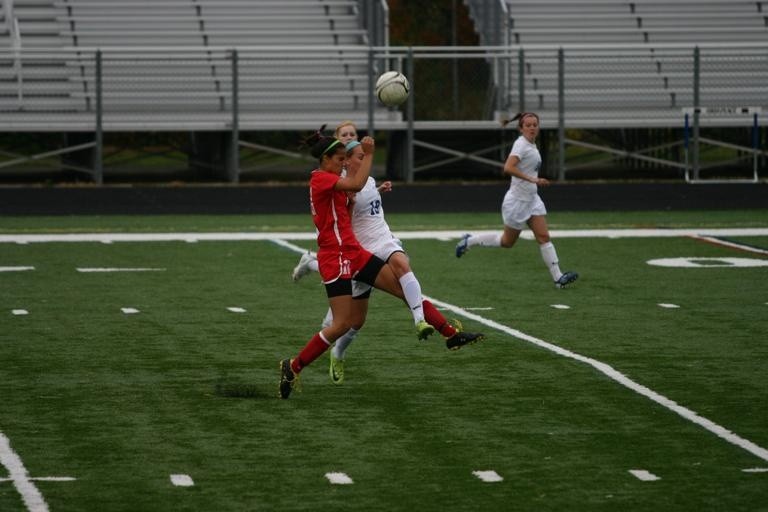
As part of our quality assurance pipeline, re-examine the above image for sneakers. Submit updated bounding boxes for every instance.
[416,320,433,340]
[291,254,315,281]
[330,347,345,385]
[278,358,294,399]
[555,273,578,289]
[456,235,469,258]
[446,333,484,350]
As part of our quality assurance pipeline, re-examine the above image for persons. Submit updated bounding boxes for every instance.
[278,124,485,400]
[455,112,578,289]
[291,121,393,329]
[329,141,435,386]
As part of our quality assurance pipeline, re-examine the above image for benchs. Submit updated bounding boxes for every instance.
[0,0,768,122]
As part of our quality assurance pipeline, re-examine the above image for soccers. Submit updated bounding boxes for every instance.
[376,71,410,107]
